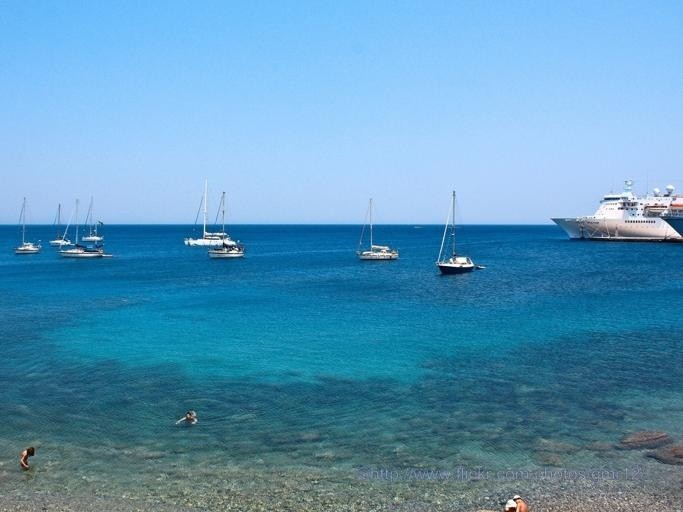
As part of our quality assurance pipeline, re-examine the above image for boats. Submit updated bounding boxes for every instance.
[641,184,683,219]
[549,173,683,242]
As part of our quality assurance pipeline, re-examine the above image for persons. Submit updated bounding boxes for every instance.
[19,446,34,470]
[512,495,528,512]
[505,498,516,512]
[175,411,197,425]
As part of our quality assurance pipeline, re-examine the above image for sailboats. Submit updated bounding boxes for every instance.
[82,188,105,242]
[14,196,42,253]
[436,190,475,274]
[59,197,113,259]
[356,198,400,260]
[48,197,73,245]
[184,179,245,257]
